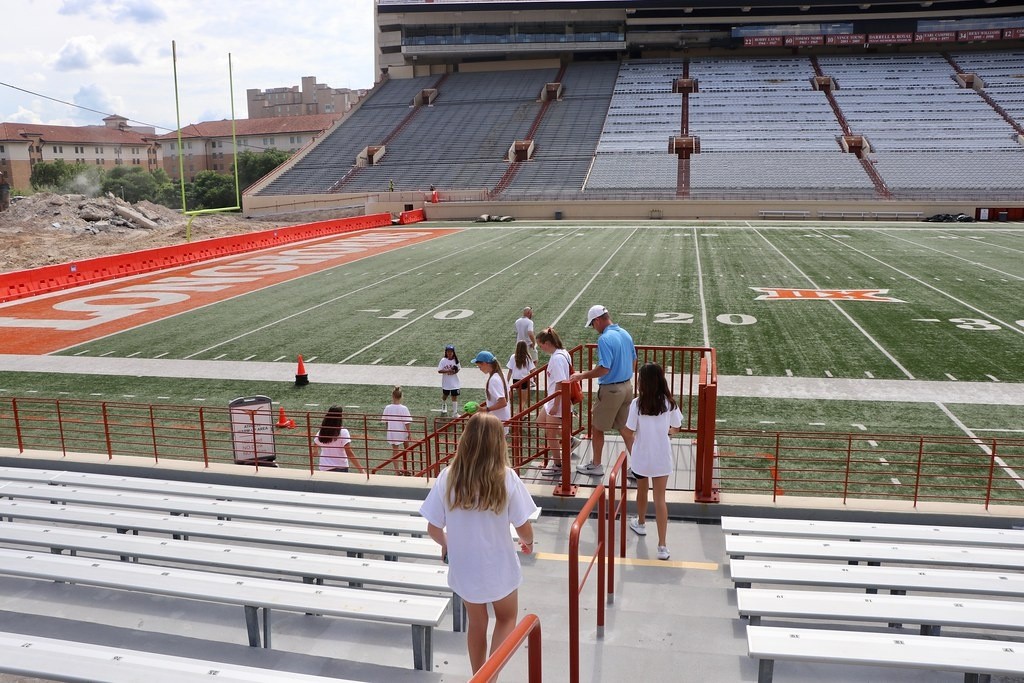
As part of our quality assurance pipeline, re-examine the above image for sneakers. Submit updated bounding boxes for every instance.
[576,459,604,475]
[571,437,581,451]
[656,545,670,559]
[631,517,646,535]
[541,464,562,475]
[627,466,636,478]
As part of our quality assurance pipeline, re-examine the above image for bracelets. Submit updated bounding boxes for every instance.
[487,407,489,412]
[526,541,533,545]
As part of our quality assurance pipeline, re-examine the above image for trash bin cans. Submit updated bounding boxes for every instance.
[555,212,562,220]
[999,212,1008,222]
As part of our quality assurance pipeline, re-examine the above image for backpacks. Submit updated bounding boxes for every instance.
[556,353,584,405]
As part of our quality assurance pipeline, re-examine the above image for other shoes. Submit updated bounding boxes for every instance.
[441,404,448,413]
[530,381,535,386]
[452,413,461,417]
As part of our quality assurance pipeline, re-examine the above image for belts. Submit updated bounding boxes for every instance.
[610,378,630,384]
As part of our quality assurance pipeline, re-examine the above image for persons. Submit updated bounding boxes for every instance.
[535,327,582,476]
[515,306,538,386]
[624,362,684,561]
[569,304,638,479]
[380,386,412,476]
[313,405,367,474]
[471,351,510,435]
[505,340,537,421]
[418,412,538,683]
[437,345,461,418]
[388,179,394,192]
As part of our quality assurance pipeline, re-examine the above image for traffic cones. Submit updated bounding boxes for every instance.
[294,354,309,386]
[275,407,289,426]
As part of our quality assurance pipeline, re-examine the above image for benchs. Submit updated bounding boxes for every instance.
[0,465,542,683]
[759,211,809,220]
[720,515,1023,683]
[873,212,925,221]
[817,211,870,221]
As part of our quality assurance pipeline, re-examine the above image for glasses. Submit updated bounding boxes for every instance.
[528,307,532,313]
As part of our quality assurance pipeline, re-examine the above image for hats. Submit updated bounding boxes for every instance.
[444,345,454,350]
[584,305,608,328]
[470,351,495,364]
